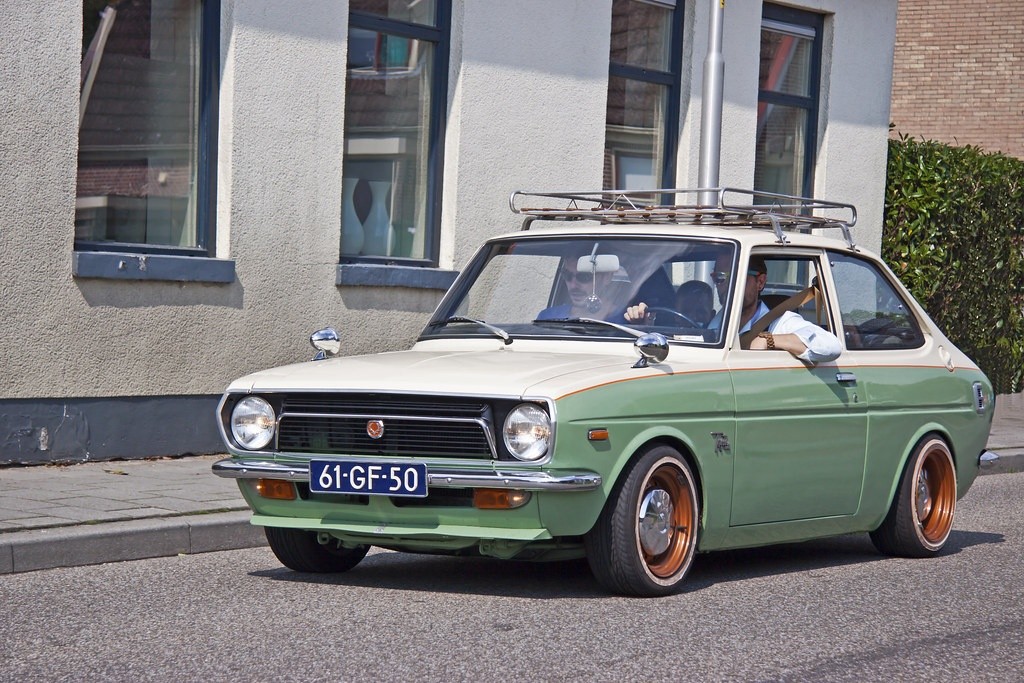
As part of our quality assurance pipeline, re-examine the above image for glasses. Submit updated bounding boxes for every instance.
[561,270,593,283]
[710,271,761,285]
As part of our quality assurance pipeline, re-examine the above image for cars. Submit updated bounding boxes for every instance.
[211,186,1001,598]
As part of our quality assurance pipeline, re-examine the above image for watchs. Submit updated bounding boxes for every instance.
[758,331,775,350]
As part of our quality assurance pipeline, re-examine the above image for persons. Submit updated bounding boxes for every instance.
[533,243,640,330]
[624,247,843,366]
[671,280,716,329]
